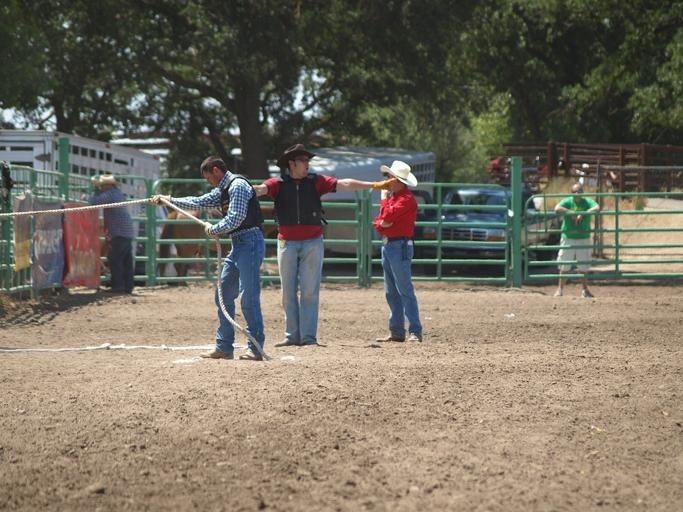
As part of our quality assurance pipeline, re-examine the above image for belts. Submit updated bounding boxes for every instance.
[386,236,412,241]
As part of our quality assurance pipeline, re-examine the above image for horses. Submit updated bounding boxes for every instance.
[156,205,277,286]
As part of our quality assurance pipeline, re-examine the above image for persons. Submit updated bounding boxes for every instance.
[87,173,136,295]
[552,182,600,299]
[149,155,268,362]
[250,141,403,348]
[371,158,425,346]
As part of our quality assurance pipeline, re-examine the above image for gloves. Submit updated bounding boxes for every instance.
[375,177,396,190]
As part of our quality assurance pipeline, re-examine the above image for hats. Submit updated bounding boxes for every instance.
[276,144,316,168]
[91,174,121,185]
[380,160,418,187]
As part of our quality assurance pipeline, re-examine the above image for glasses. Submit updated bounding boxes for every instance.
[294,158,311,163]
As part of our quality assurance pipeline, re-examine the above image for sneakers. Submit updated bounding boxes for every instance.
[582,290,594,298]
[554,290,563,296]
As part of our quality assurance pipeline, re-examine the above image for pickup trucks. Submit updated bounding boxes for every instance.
[419,176,559,275]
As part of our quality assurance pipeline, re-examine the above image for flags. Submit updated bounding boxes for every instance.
[61,200,102,292]
[12,193,33,270]
[30,196,65,291]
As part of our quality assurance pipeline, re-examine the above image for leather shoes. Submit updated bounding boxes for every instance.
[274,339,301,346]
[199,350,234,359]
[239,352,263,360]
[376,335,405,342]
[408,333,422,343]
